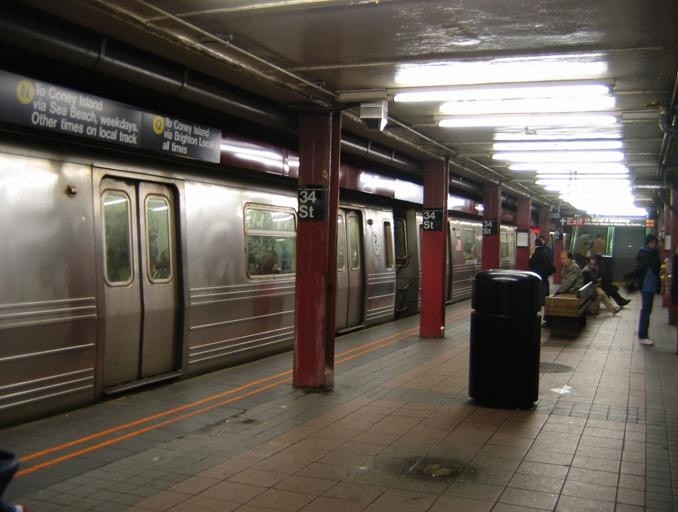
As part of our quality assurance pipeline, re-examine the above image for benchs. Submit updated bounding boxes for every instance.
[543,286,597,337]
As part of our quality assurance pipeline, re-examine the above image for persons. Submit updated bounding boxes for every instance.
[527,237,552,307]
[541,232,631,328]
[634,233,662,346]
[538,235,553,296]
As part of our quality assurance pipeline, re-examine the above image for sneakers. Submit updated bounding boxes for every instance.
[612,297,631,314]
[639,338,654,344]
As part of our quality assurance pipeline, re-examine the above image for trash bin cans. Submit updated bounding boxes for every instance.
[469,269,545,410]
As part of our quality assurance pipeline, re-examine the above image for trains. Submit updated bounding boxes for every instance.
[1,122,570,453]
